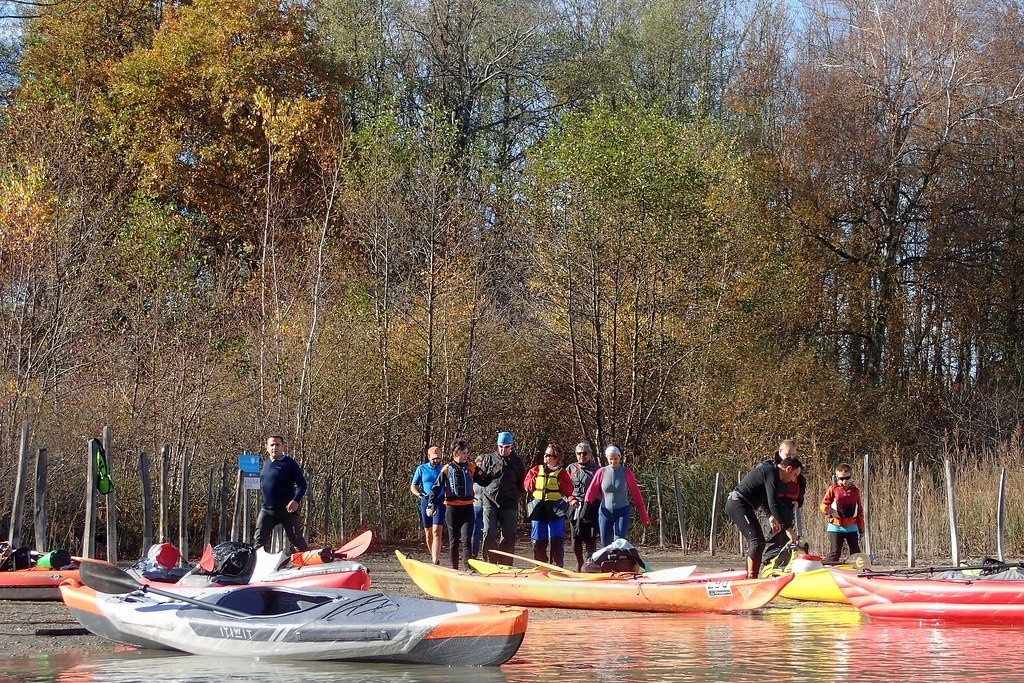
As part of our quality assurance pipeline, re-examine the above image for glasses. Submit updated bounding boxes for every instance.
[577,452,590,456]
[837,476,851,481]
[544,452,554,458]
[500,443,511,449]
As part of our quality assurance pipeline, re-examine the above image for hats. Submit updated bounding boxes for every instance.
[498,432,514,444]
[428,446,442,459]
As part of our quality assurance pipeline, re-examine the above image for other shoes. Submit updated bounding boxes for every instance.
[575,560,585,572]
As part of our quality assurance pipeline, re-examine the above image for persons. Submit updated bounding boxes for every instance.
[410,447,446,565]
[565,442,603,573]
[481,432,527,566]
[580,443,651,548]
[523,444,574,568]
[725,456,803,579]
[754,439,804,575]
[471,454,485,559]
[253,434,311,553]
[820,463,863,562]
[0,541,12,567]
[425,440,503,572]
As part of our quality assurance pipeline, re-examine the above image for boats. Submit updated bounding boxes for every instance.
[829,564,1024,627]
[392,548,796,614]
[0,540,117,600]
[762,533,874,605]
[125,556,371,592]
[56,576,531,668]
[467,557,750,582]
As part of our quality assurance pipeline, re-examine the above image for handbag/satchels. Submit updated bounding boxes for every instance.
[527,499,544,520]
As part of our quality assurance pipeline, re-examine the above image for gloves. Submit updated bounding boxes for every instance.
[491,461,502,476]
[426,502,436,518]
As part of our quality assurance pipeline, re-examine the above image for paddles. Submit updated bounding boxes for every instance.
[489,549,613,578]
[334,529,373,560]
[175,542,215,587]
[78,560,250,617]
[822,552,872,570]
